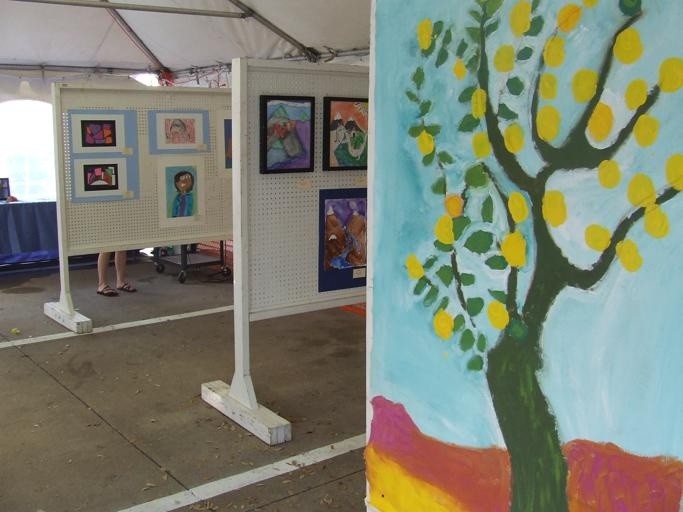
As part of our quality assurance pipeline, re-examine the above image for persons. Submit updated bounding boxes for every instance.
[94,250,137,298]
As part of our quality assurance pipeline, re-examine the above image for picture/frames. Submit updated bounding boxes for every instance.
[322,96,370,171]
[71,114,125,153]
[157,156,205,227]
[148,109,211,155]
[318,187,367,293]
[259,94,315,174]
[74,157,128,198]
[216,109,232,179]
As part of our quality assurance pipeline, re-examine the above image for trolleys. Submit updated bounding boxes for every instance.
[150,240,231,283]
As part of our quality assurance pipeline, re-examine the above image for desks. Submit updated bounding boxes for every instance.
[0,200,145,274]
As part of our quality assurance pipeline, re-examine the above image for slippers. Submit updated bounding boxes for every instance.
[117,283,136,292]
[97,285,119,297]
